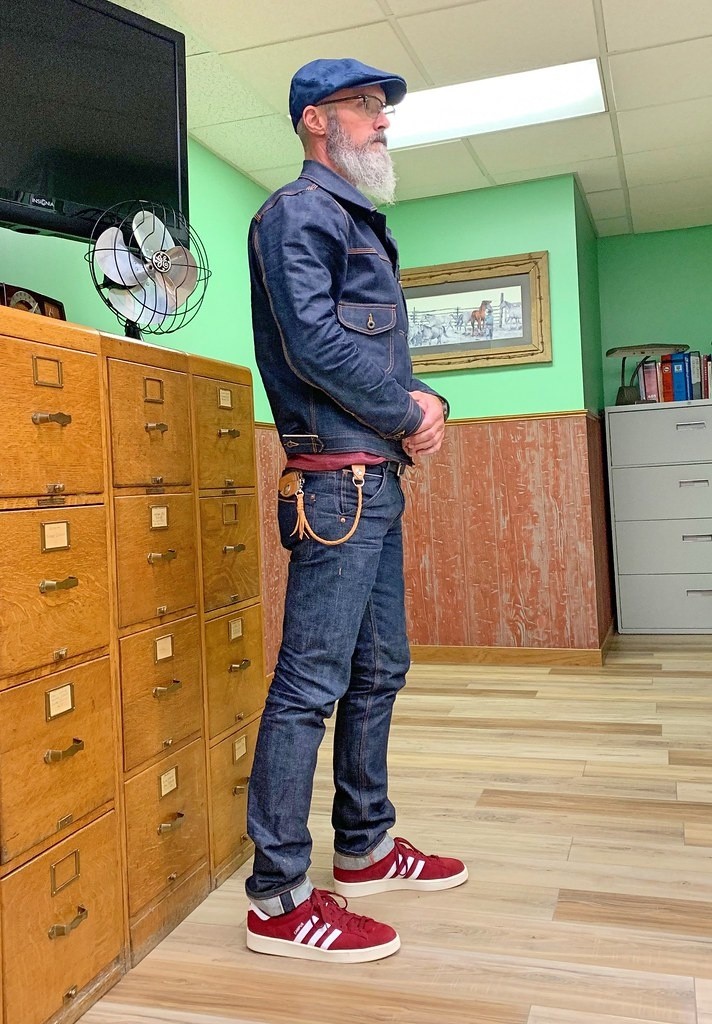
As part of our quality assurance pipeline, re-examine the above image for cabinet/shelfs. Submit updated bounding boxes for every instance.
[97,328,211,973]
[604,399,712,634]
[0,305,127,1024]
[187,354,269,890]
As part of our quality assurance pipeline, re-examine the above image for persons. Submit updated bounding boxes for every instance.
[239,56,466,964]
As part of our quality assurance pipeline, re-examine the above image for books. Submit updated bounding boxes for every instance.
[637,349,712,403]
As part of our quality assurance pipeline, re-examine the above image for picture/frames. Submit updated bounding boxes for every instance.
[397,250,554,375]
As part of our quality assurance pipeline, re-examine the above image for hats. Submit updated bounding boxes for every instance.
[289,59,407,134]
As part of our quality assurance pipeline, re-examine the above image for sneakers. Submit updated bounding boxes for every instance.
[247,889,402,964]
[332,836,468,898]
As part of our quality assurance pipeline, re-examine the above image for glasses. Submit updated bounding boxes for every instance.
[316,95,396,120]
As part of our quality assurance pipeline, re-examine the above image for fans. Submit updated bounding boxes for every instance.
[85,198,212,340]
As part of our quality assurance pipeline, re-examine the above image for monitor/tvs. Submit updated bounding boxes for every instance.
[0,0,190,256]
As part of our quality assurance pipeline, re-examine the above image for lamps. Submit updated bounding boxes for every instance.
[605,343,690,406]
